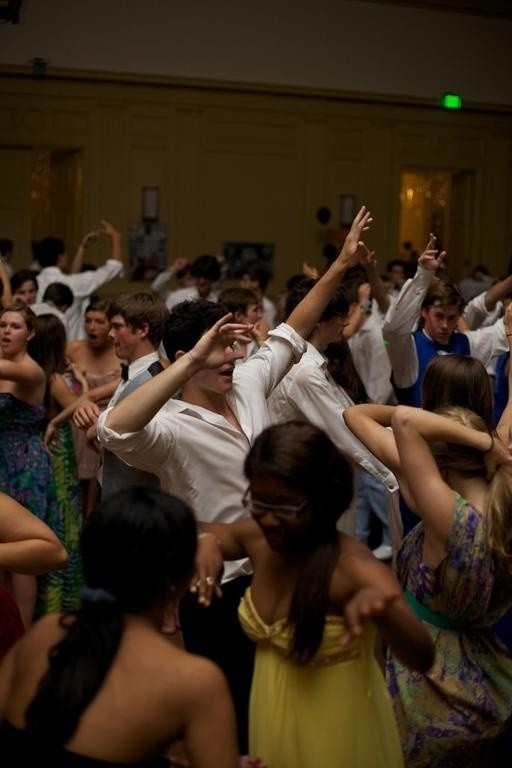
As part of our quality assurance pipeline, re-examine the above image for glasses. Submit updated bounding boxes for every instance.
[242,486,308,520]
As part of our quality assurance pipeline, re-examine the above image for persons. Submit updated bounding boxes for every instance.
[2,191,511,768]
